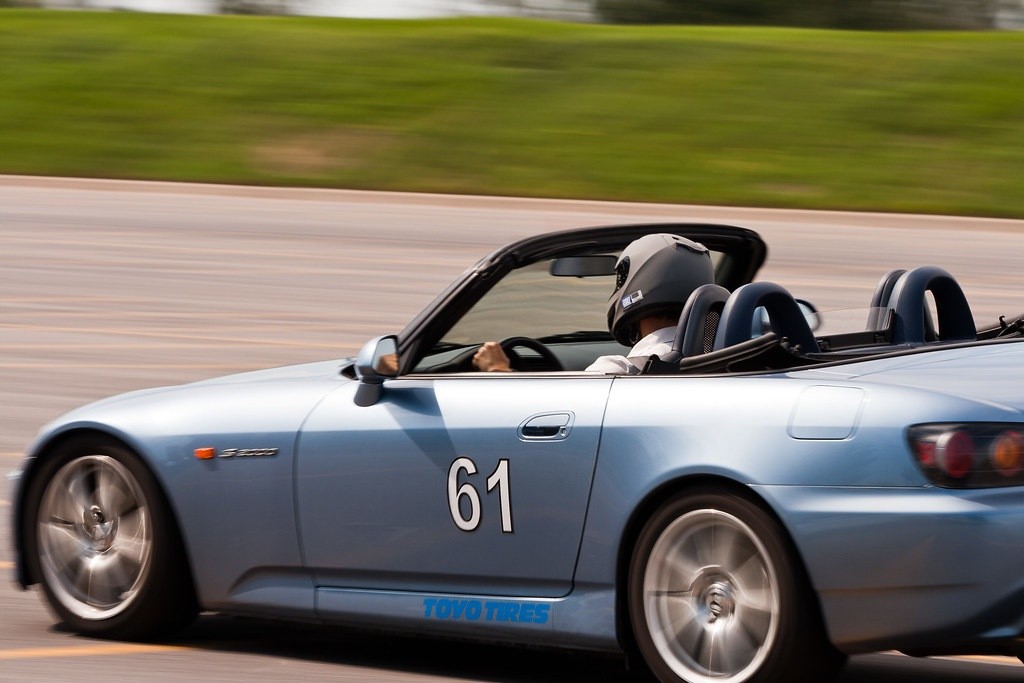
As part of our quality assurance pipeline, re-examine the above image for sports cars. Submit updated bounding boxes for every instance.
[8,220,1024,683]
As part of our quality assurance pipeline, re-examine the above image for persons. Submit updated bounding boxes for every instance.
[473,232,717,374]
[377,353,398,374]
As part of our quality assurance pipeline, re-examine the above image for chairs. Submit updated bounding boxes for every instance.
[657,285,731,363]
[863,267,938,342]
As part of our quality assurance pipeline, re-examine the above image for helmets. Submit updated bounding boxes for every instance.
[606,233,716,347]
